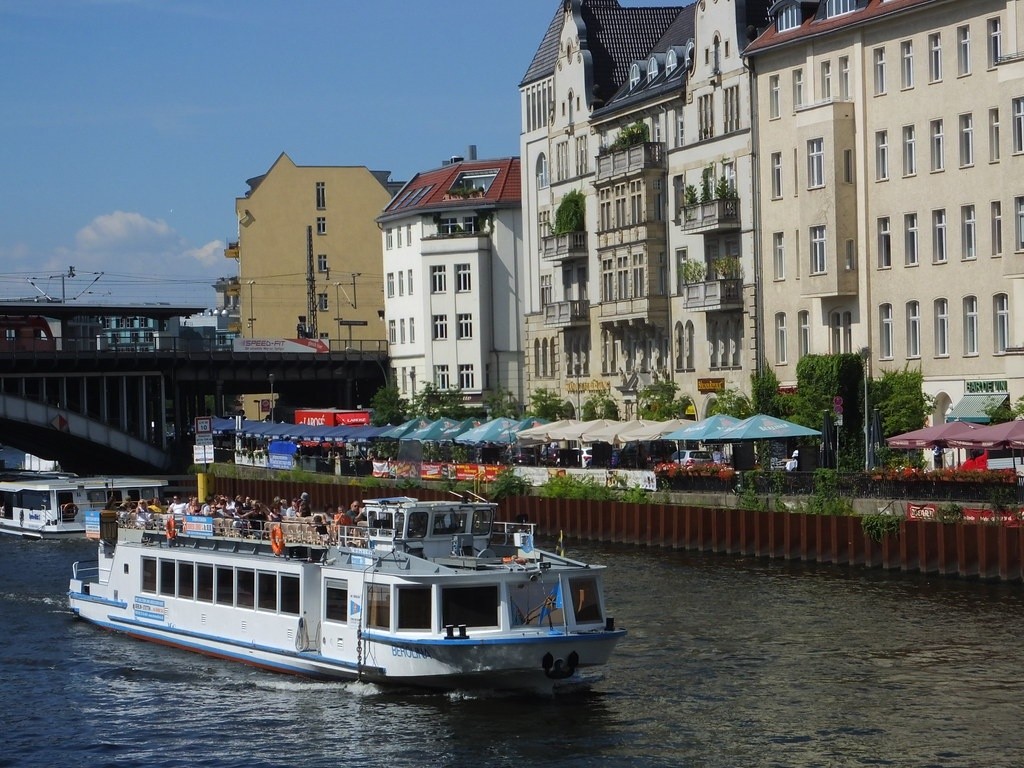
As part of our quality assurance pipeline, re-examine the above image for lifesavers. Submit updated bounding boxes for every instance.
[271,526,284,555]
[63,504,79,519]
[166,518,176,539]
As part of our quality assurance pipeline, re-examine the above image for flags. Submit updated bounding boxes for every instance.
[538,579,563,625]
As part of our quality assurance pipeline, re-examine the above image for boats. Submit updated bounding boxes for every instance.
[66,475,626,693]
[0,479,170,539]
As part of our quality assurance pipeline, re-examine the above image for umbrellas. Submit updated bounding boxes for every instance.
[885,418,1024,470]
[201,413,823,466]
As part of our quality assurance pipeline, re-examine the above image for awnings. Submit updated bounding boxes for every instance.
[947,395,1007,423]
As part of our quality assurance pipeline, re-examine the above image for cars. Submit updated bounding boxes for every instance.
[673,451,713,467]
[555,445,593,468]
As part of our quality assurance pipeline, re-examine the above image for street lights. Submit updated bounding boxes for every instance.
[247,281,256,340]
[575,365,582,421]
[268,374,276,423]
[345,347,388,390]
[863,348,869,472]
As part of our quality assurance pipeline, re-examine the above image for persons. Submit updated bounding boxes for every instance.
[105,491,367,560]
